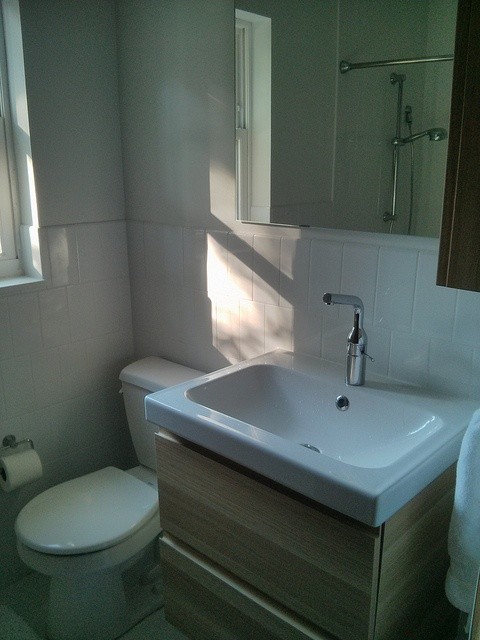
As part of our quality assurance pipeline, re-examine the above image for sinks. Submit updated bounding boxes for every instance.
[145,349,479,531]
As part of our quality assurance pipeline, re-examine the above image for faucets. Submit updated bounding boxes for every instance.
[322,292,375,386]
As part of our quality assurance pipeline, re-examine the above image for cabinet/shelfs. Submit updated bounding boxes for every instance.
[154,426,456,639]
[437,0,480,294]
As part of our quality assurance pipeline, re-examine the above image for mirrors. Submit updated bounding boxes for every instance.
[234,1,458,238]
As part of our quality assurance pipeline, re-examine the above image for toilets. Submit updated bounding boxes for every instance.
[14,356,208,640]
